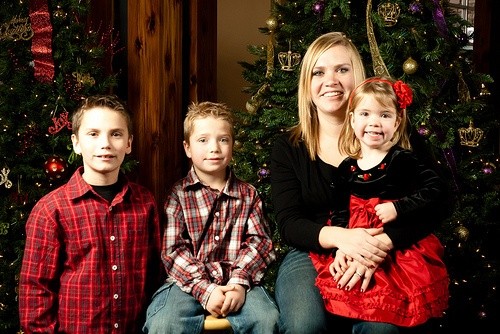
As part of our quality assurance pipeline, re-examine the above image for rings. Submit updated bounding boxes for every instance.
[355,271,362,278]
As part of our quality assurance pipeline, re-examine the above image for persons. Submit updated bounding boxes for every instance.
[18,94,165,334]
[140,102,281,334]
[269,31,456,334]
[308,76,451,328]
